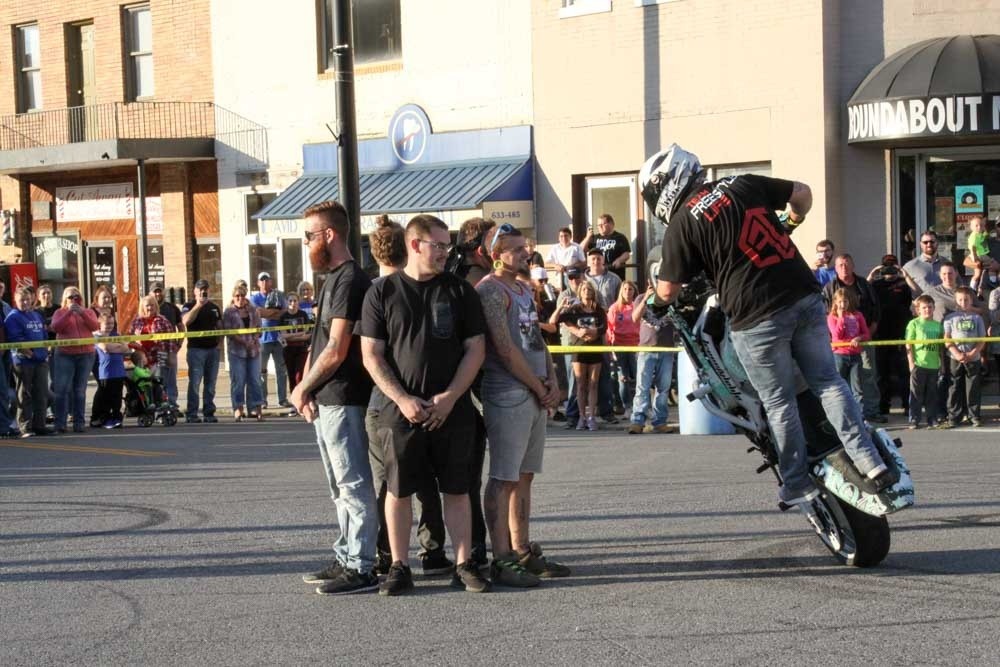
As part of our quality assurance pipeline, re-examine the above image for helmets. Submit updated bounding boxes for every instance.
[638,143,708,227]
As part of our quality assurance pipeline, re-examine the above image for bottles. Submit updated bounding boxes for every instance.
[544,284,556,302]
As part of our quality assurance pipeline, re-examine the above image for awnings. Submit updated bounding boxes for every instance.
[250,161,532,219]
[847,32,1000,148]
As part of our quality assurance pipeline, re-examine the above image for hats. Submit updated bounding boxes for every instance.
[530,267,548,279]
[150,281,162,291]
[257,272,272,281]
[195,280,210,289]
[566,266,579,275]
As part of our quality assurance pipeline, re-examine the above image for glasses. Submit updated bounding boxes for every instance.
[418,240,453,253]
[922,240,936,245]
[304,228,327,241]
[65,294,81,300]
[490,223,511,252]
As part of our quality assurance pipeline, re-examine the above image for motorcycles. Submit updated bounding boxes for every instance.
[644,214,915,568]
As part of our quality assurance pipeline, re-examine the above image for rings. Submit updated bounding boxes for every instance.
[436,416,441,421]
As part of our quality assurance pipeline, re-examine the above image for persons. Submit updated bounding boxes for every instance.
[181,278,225,426]
[809,215,1000,430]
[285,200,572,597]
[0,279,182,438]
[225,266,317,424]
[521,210,681,436]
[636,143,899,511]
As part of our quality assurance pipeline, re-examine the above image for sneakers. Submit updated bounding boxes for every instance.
[776,483,819,505]
[862,463,887,484]
[303,541,572,595]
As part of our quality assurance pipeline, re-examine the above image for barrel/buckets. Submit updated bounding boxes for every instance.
[677,343,736,435]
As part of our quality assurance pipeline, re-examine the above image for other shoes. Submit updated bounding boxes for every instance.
[0,405,298,440]
[863,405,981,429]
[553,388,674,434]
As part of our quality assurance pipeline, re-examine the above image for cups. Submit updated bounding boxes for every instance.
[277,334,288,347]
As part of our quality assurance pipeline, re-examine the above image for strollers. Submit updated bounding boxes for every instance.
[118,341,177,428]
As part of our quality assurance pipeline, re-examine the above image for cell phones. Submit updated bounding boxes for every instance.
[880,267,897,274]
[203,292,207,298]
